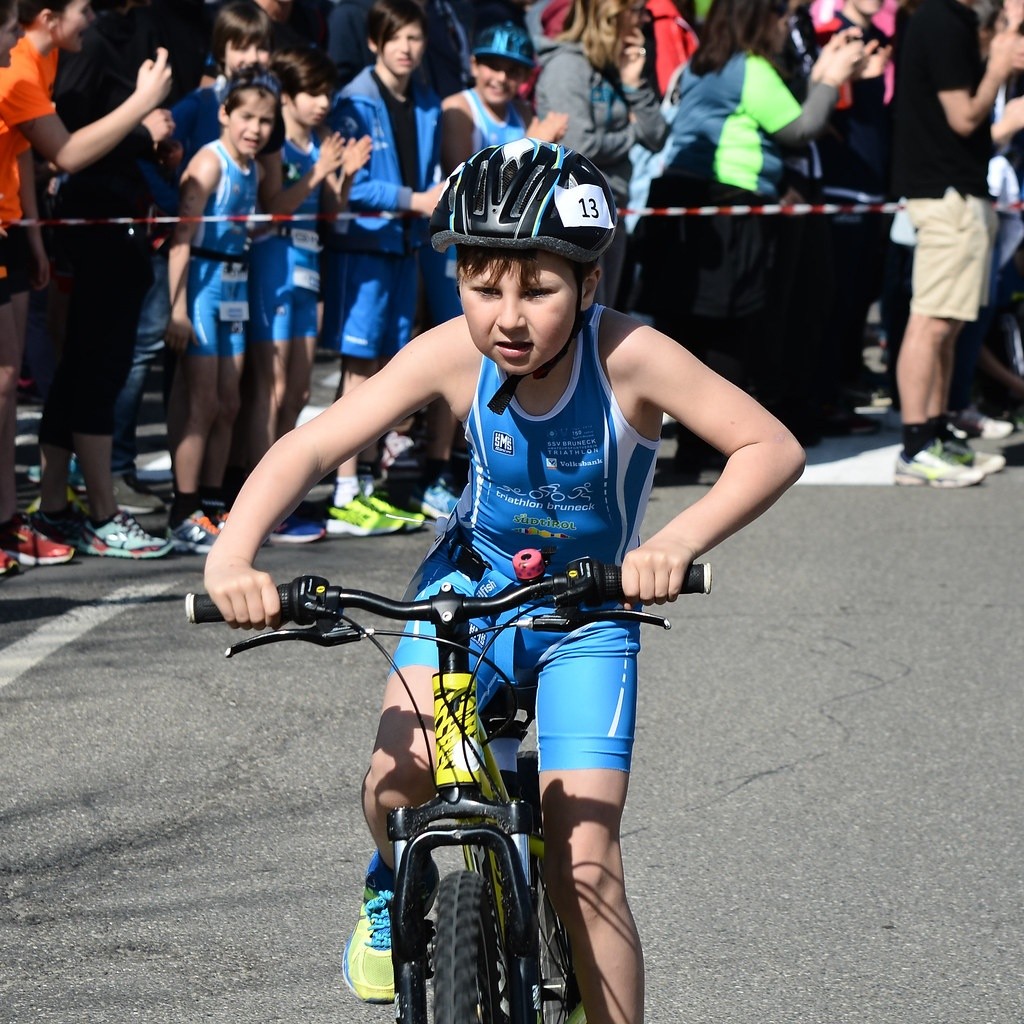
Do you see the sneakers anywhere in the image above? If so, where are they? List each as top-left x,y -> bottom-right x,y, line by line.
935,433 -> 1006,474
203,510 -> 268,545
438,476 -> 462,499
31,511 -> 89,553
325,493 -> 406,537
166,508 -> 221,554
0,522 -> 74,565
361,495 -> 426,530
408,482 -> 462,519
341,850 -> 438,1004
267,514 -> 325,543
0,549 -> 18,575
69,467 -> 163,512
76,512 -> 174,560
894,438 -> 983,489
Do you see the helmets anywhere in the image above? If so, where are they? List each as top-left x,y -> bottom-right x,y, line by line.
429,137 -> 617,263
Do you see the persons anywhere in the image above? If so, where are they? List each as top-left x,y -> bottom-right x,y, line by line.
201,140 -> 808,1024
0,0 -> 1024,577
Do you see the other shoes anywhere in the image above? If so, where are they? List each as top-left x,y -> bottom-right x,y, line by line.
663,443 -> 727,487
948,402 -> 1014,437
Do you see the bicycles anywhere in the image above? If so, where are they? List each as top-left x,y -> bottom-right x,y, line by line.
184,548 -> 711,1024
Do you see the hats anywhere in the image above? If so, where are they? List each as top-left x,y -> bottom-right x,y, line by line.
470,22 -> 537,71
